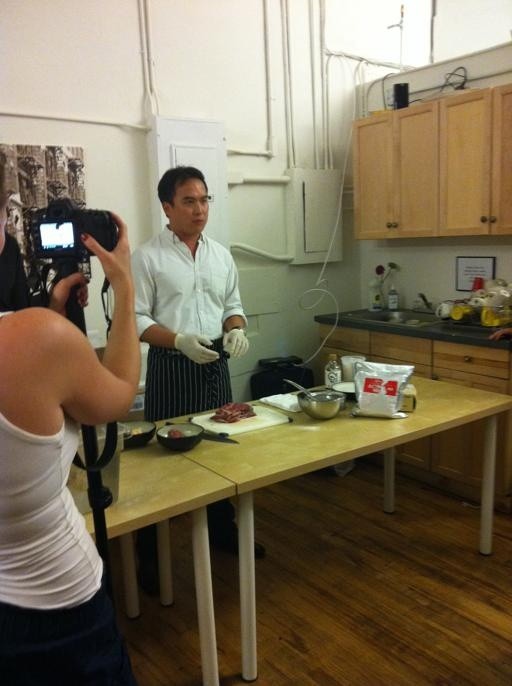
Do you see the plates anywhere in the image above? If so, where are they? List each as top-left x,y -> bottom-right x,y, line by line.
332,383 -> 356,395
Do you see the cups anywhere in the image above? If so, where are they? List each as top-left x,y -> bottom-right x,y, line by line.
341,356 -> 366,382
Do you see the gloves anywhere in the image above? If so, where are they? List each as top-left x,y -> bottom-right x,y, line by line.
222,327 -> 249,362
175,333 -> 219,365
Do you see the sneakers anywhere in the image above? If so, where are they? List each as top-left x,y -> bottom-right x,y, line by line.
212,533 -> 264,558
137,558 -> 161,599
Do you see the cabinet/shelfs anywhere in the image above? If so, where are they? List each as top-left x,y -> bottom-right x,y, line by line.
370,331 -> 431,470
431,332 -> 511,496
318,325 -> 370,387
351,100 -> 439,240
439,83 -> 512,236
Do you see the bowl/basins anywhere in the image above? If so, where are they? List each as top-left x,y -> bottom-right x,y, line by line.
297,391 -> 344,420
156,425 -> 204,451
122,422 -> 155,446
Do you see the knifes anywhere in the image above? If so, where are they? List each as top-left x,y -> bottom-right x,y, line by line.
166,422 -> 239,444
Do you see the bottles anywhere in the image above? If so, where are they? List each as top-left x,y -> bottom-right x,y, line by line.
387,284 -> 400,310
324,354 -> 342,390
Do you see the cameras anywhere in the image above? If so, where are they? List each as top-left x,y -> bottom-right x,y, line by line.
30,200 -> 116,257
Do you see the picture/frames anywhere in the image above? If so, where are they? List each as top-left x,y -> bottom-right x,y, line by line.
455,256 -> 495,291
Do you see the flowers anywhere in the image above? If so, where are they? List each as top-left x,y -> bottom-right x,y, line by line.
376,261 -> 397,282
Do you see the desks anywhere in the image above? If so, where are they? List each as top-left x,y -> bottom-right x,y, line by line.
82,439 -> 236,685
182,385 -> 512,682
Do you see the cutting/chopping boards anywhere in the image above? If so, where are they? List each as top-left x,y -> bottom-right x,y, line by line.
189,406 -> 294,437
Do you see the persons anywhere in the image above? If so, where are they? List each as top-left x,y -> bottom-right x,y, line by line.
106,166 -> 266,583
1,148 -> 141,686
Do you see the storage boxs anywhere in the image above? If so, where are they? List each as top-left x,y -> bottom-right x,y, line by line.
67,421 -> 127,516
